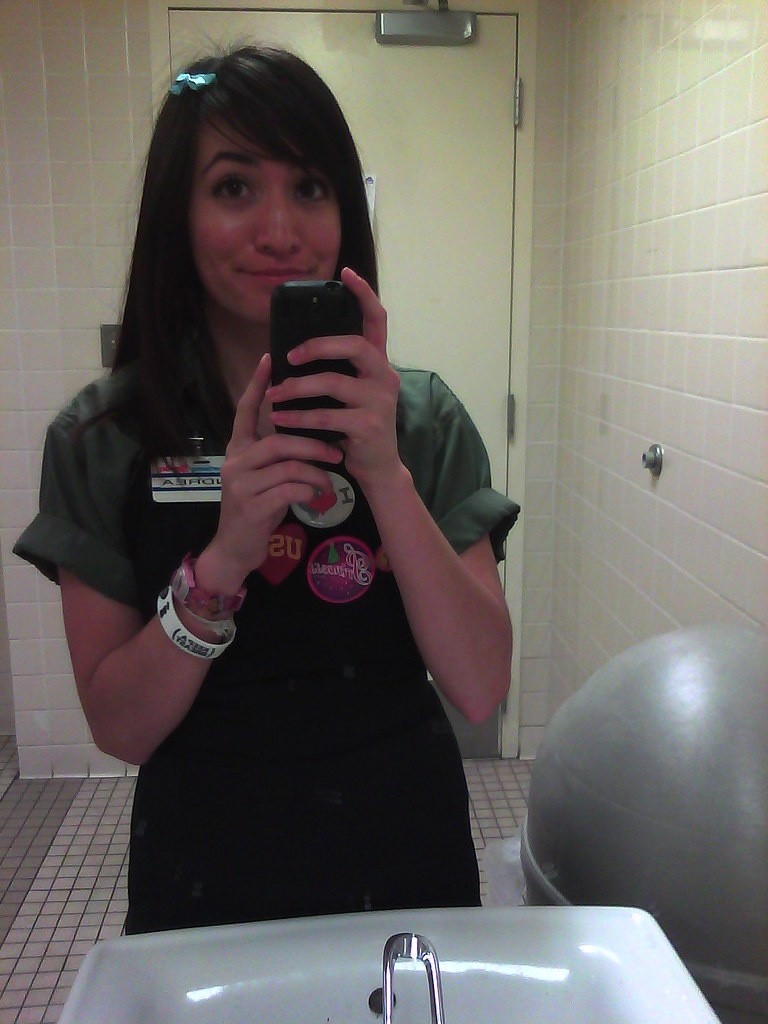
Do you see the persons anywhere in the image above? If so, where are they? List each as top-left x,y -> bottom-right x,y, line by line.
13,34 -> 522,934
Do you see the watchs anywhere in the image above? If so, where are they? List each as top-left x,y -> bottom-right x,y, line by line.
175,553 -> 246,621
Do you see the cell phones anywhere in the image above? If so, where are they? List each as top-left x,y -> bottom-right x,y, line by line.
270,279 -> 358,441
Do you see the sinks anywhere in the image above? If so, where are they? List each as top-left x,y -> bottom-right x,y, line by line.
57,906 -> 720,1024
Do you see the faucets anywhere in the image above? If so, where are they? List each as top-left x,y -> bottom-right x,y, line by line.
384,934 -> 445,1024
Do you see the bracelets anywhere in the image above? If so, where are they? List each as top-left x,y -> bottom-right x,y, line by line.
157,586 -> 237,660
